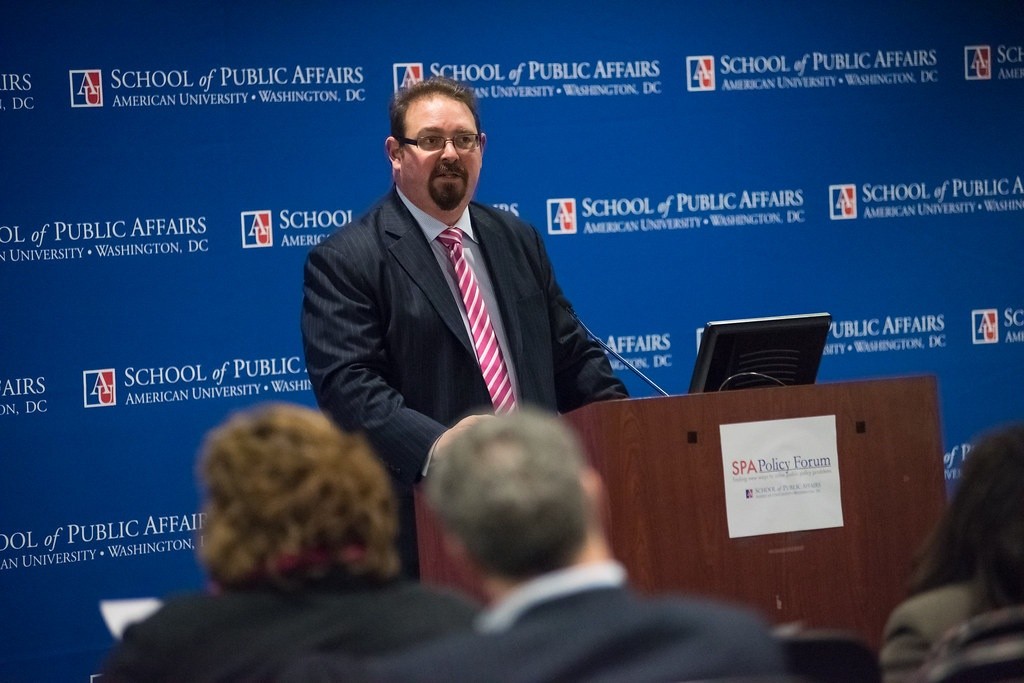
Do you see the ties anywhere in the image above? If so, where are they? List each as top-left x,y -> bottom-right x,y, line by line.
437,227 -> 517,417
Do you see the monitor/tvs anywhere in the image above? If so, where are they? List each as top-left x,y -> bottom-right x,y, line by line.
688,312 -> 832,393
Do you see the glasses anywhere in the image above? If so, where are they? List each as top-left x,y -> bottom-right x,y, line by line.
400,133 -> 482,150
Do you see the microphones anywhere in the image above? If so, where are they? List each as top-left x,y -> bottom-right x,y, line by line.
556,295 -> 671,397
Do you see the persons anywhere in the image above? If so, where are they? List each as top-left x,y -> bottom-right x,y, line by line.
99,407 -> 474,683
300,80 -> 630,593
880,428 -> 1024,683
278,399 -> 790,683
